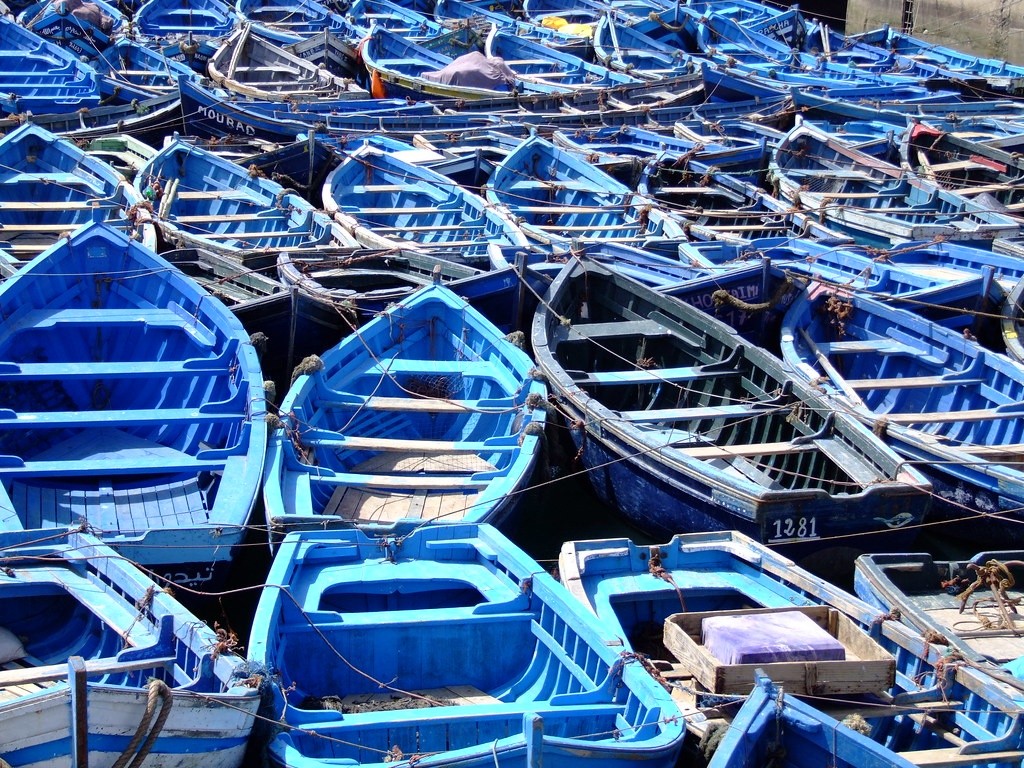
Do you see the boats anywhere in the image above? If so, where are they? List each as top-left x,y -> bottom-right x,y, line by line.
0,201 -> 270,566
853,548 -> 1024,693
261,280 -> 549,566
245,520 -> 687,767
766,283 -> 1024,525
530,249 -> 934,562
706,664 -> 919,768
557,529 -> 1024,768
0,521 -> 262,766
0,0 -> 1024,339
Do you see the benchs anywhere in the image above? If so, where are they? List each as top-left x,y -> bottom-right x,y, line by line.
788,170 -> 965,225
1,310 -> 253,482
0,0 -> 1024,214
503,179 -> 665,242
340,180 -> 503,248
558,310 -> 835,459
809,326 -> 1024,458
282,356 -> 534,490
0,552 -> 1024,768
0,166 -> 135,231
172,182 -> 333,254
656,185 -> 791,232
